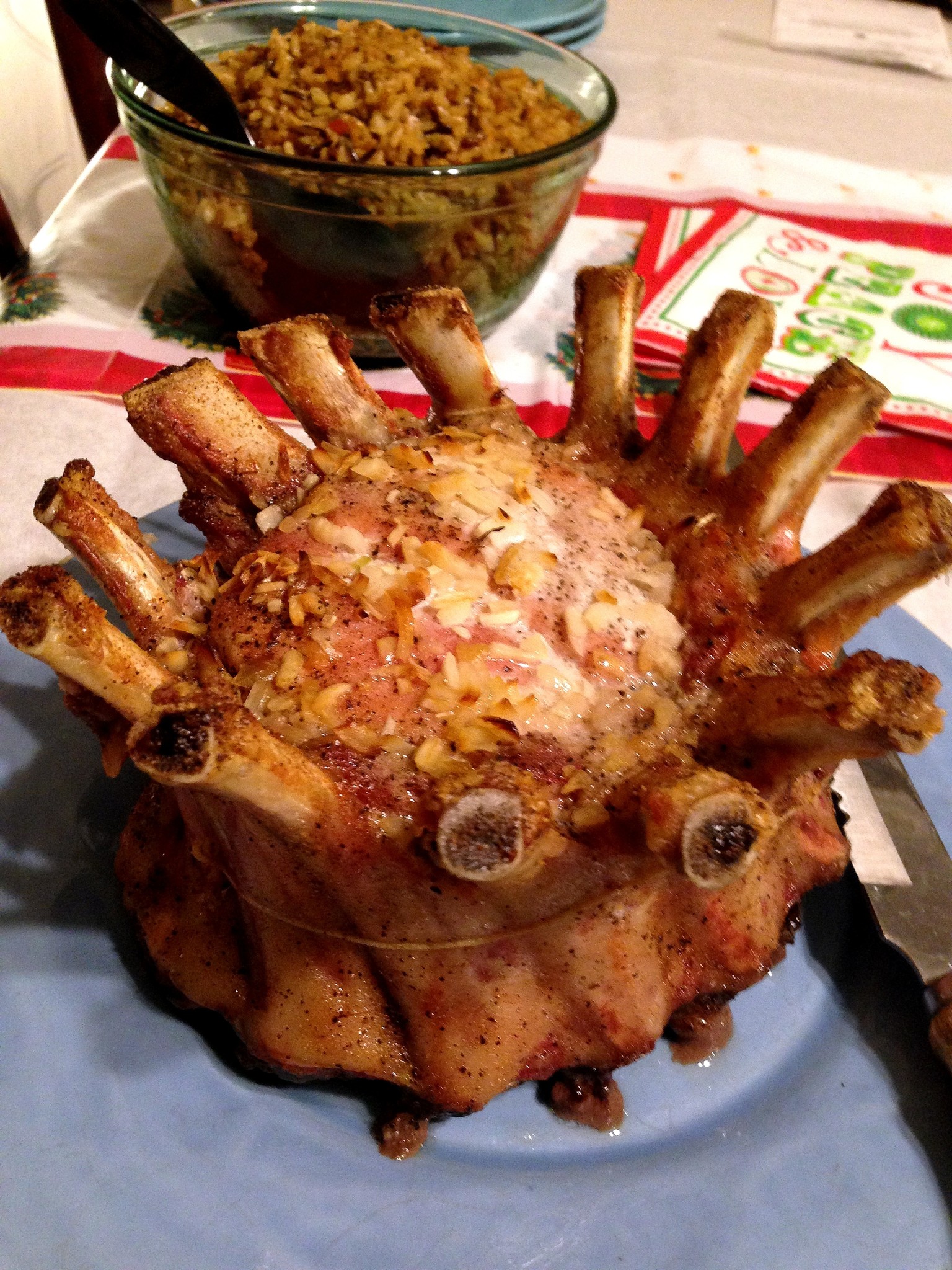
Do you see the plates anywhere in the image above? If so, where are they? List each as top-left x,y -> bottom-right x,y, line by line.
2,472 -> 952,1269
237,1 -> 608,68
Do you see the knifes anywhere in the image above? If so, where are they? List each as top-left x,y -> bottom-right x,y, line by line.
816,636 -> 952,1064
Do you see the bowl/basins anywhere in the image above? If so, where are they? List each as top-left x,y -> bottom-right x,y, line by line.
106,2 -> 624,371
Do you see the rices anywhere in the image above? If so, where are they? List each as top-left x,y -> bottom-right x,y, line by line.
129,10 -> 599,340
